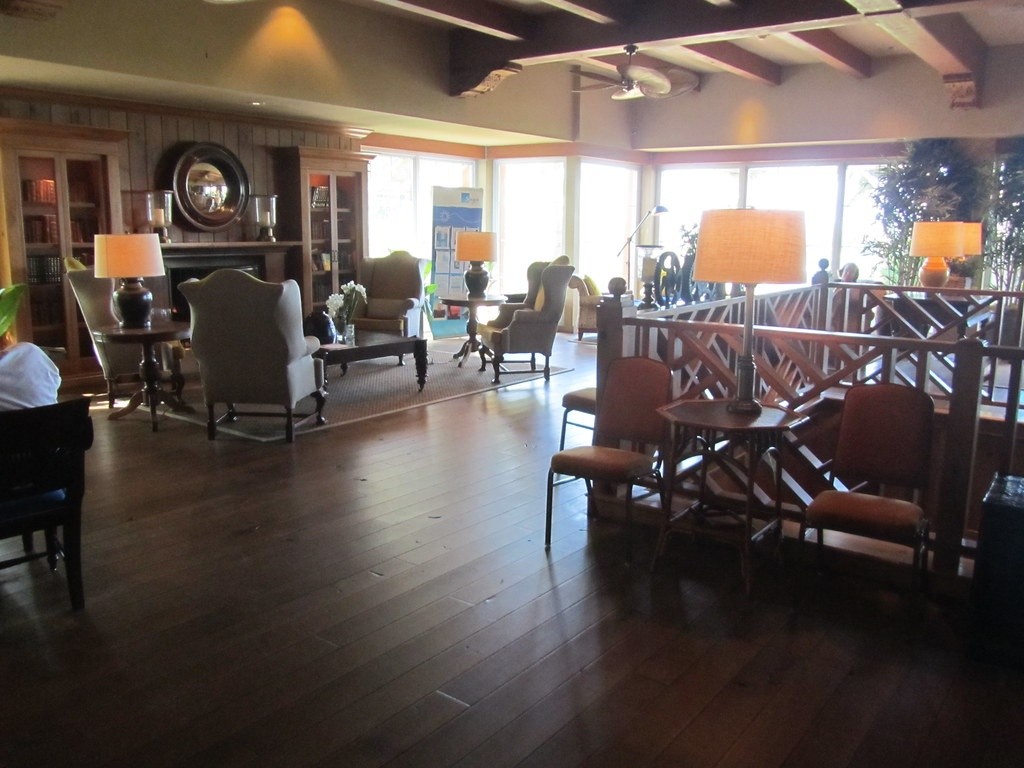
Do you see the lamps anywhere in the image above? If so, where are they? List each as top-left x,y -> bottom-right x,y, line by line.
146,189 -> 173,242
910,220 -> 983,302
457,232 -> 496,298
249,193 -> 279,243
692,208 -> 808,417
92,233 -> 165,331
619,205 -> 670,290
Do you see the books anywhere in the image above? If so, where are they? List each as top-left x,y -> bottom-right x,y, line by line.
311,185 -> 348,240
312,247 -> 354,302
20,179 -> 99,284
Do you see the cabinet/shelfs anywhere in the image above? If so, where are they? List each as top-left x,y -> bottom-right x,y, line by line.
0,118 -> 129,397
296,144 -> 376,320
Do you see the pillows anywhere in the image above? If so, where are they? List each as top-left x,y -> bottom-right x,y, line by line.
583,273 -> 600,295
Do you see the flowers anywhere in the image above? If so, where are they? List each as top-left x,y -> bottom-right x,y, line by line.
327,282 -> 366,316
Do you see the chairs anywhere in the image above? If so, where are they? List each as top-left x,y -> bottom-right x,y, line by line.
0,398 -> 93,613
791,379 -> 942,636
178,269 -> 326,443
544,358 -> 674,557
353,249 -> 433,365
476,254 -> 576,385
568,274 -> 637,342
61,255 -> 186,407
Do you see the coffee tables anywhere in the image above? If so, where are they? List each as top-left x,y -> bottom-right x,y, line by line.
316,330 -> 429,393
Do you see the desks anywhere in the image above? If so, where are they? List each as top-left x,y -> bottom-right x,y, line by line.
657,398 -> 811,608
92,322 -> 194,432
441,298 -> 495,369
884,293 -> 999,403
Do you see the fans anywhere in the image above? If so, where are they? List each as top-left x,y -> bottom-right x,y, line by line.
566,47 -> 701,100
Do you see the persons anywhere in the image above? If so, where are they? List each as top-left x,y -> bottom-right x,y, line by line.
0,328 -> 66,508
832,261 -> 859,304
